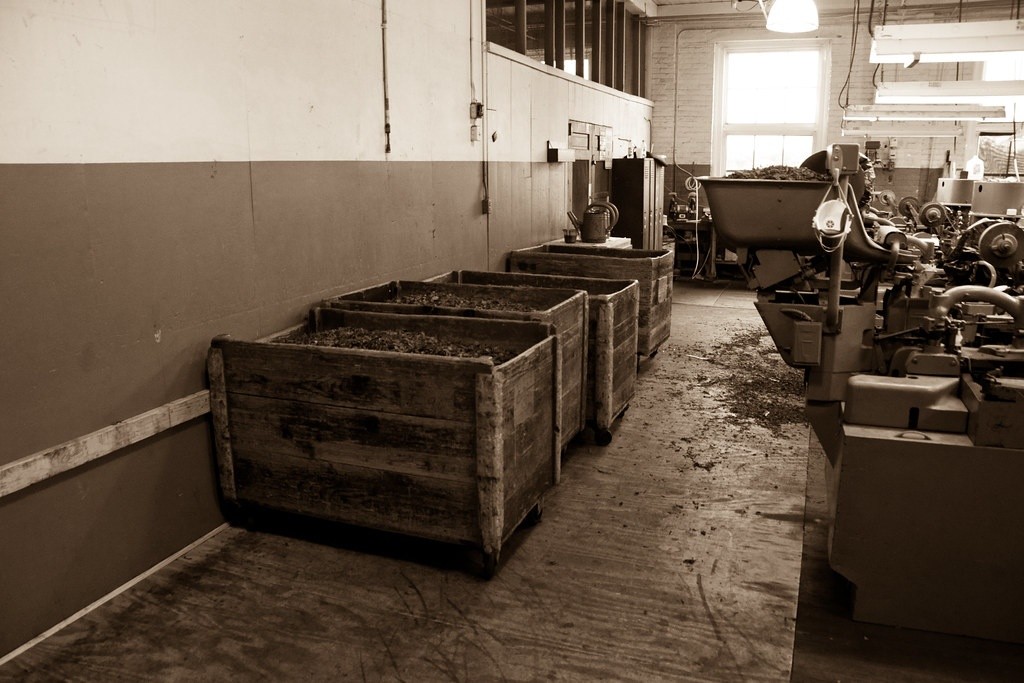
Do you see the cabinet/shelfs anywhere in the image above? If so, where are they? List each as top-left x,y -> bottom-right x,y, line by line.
613,158 -> 665,251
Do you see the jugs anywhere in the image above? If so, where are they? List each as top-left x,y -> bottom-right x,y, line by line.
567,202 -> 619,243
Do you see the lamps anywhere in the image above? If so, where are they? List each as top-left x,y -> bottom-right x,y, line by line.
875,80 -> 1024,108
869,20 -> 1024,63
767,0 -> 819,33
843,105 -> 1005,120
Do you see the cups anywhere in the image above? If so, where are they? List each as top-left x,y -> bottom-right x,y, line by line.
563,229 -> 578,244
